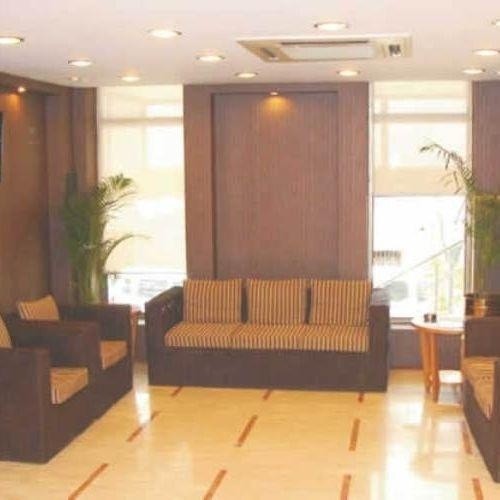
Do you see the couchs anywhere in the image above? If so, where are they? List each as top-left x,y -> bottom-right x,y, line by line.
462,316 -> 500,483
144,278 -> 390,391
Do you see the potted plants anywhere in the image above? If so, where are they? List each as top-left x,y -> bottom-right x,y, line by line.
418,141 -> 500,317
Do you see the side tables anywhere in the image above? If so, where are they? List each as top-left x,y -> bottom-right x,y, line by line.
409,314 -> 465,403
129,308 -> 142,365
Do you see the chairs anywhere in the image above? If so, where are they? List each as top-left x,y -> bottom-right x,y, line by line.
0,293 -> 134,464
